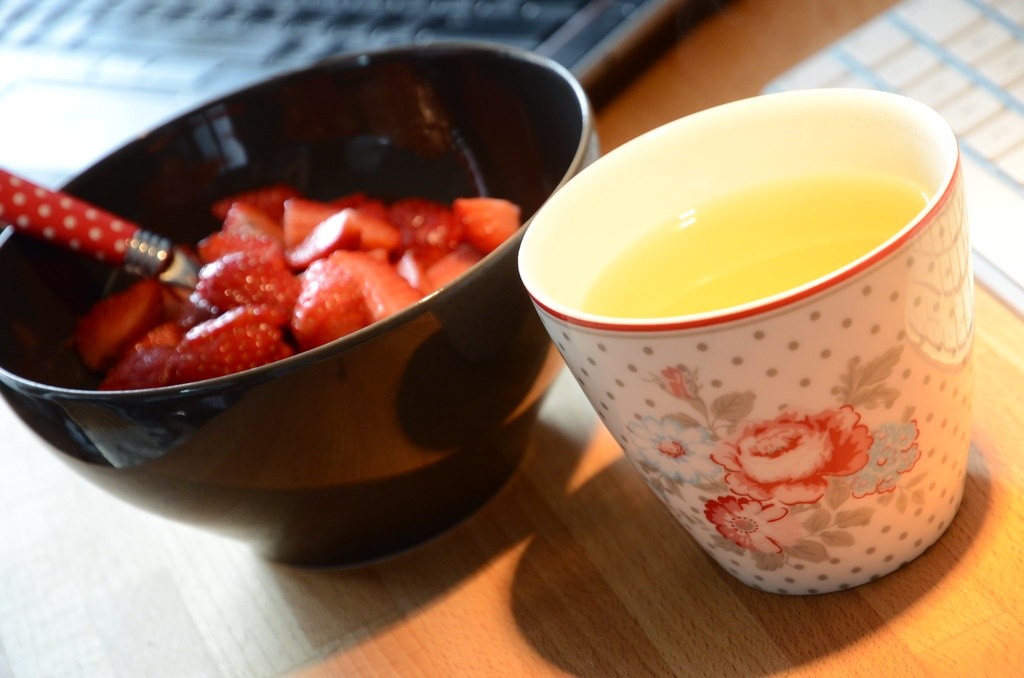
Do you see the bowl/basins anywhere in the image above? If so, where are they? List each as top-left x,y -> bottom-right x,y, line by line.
0,43 -> 600,570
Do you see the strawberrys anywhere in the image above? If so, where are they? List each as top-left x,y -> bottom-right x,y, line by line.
71,182 -> 520,388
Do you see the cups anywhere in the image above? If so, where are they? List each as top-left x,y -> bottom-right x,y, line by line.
518,82 -> 970,593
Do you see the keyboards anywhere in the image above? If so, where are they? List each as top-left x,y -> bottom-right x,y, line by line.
0,0 -> 734,198
